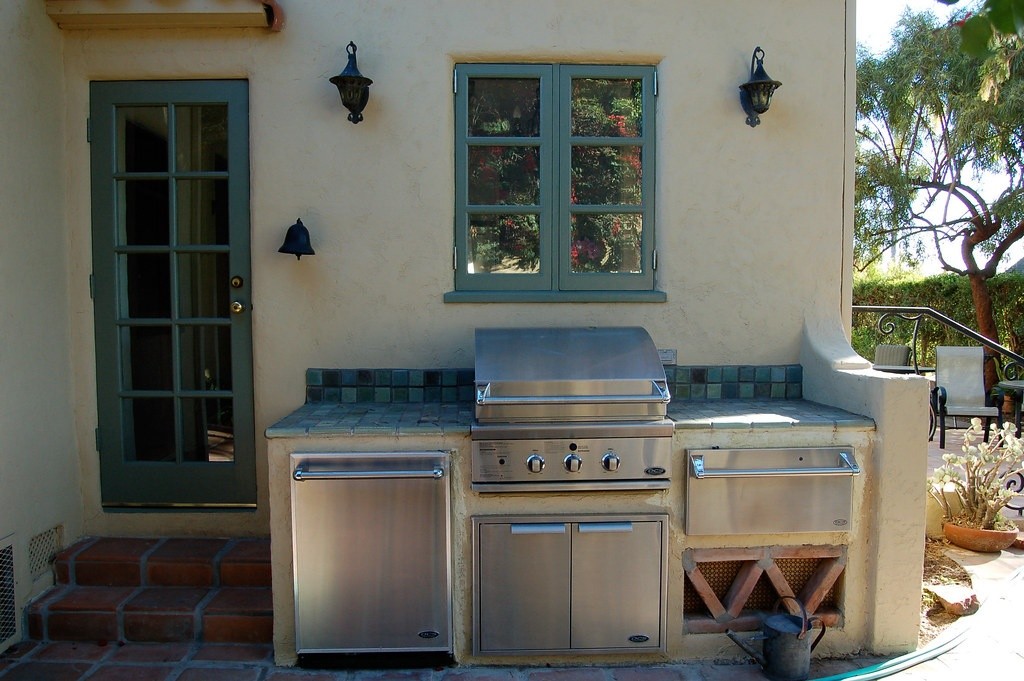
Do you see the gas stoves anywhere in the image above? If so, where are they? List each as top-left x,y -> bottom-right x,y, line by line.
471,328 -> 672,491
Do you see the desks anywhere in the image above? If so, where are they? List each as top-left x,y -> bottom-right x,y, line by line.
998,380 -> 1024,439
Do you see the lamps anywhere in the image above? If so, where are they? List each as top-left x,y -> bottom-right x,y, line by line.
277,218 -> 316,261
328,41 -> 373,124
738,47 -> 782,128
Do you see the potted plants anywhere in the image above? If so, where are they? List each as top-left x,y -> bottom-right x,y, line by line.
991,358 -> 1015,415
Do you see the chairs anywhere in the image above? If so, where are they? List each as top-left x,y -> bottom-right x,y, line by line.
875,344 -> 912,366
931,346 -> 1005,449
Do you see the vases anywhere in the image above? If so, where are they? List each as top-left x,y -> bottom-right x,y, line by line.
927,478 -> 1019,553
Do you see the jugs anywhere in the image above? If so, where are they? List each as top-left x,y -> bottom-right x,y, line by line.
726,596 -> 826,680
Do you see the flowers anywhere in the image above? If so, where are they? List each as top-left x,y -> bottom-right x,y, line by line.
926,418 -> 1024,529
